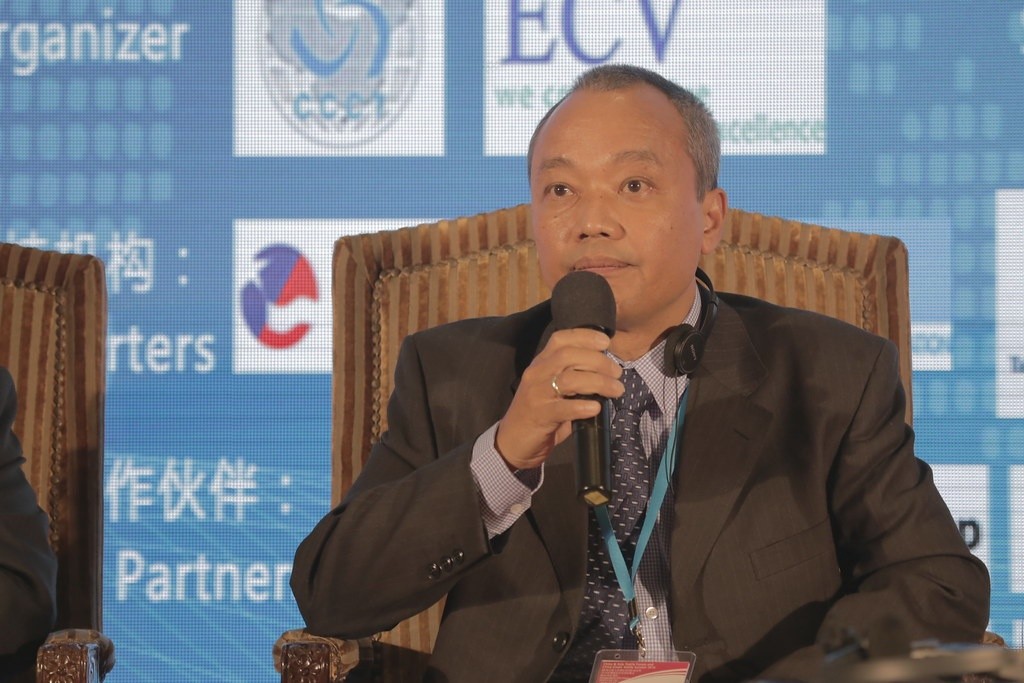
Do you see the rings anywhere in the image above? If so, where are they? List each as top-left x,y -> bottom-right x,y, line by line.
552,371 -> 563,396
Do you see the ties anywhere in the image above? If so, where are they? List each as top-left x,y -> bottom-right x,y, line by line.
561,366 -> 655,671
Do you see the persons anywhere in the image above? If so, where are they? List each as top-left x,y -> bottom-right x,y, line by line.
0,363 -> 56,683
293,65 -> 990,683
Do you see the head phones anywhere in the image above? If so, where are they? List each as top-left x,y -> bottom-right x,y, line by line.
665,267 -> 719,377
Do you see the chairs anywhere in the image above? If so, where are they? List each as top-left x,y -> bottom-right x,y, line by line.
0,243 -> 116,683
274,205 -> 1007,683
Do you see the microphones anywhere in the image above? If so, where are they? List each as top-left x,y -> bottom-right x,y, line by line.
551,270 -> 616,506
